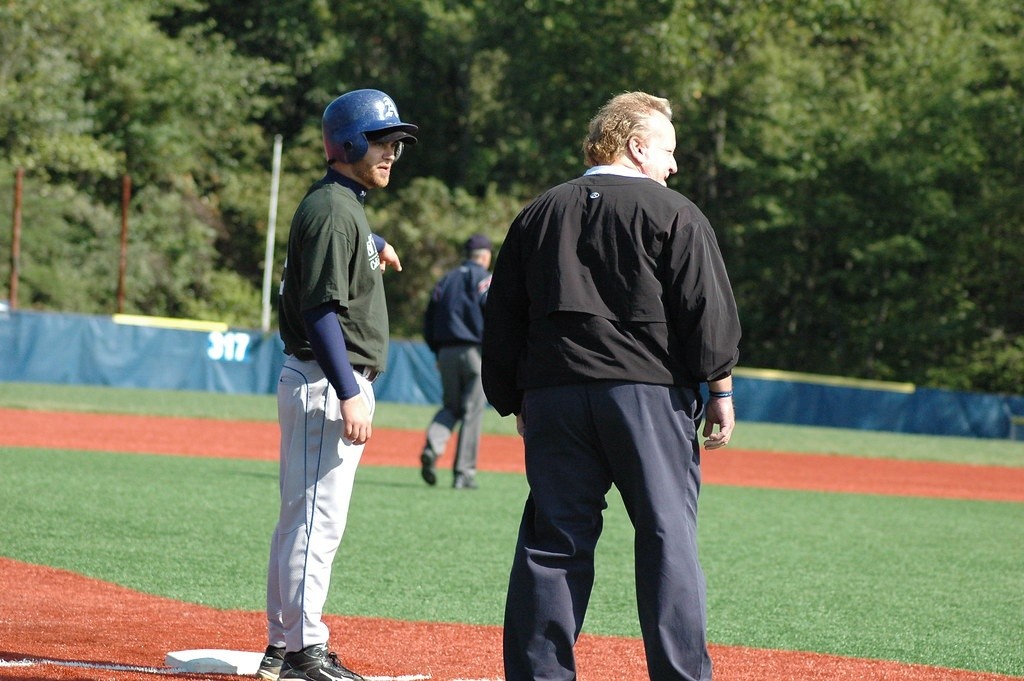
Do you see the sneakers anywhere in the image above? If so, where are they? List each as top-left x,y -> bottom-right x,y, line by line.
278,642 -> 366,681
256,645 -> 288,681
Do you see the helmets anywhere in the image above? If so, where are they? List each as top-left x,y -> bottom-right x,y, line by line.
322,90 -> 419,164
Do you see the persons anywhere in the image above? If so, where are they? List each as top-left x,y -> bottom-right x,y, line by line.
257,89 -> 417,680
482,92 -> 742,680
420,235 -> 493,490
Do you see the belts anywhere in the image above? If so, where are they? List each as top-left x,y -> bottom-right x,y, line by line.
286,339 -> 377,381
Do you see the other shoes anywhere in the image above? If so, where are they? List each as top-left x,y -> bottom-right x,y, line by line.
453,471 -> 479,487
420,448 -> 437,484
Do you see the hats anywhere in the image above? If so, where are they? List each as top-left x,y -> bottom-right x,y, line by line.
368,128 -> 418,146
464,233 -> 491,250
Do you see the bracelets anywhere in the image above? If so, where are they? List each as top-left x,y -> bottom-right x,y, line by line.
710,391 -> 734,397
